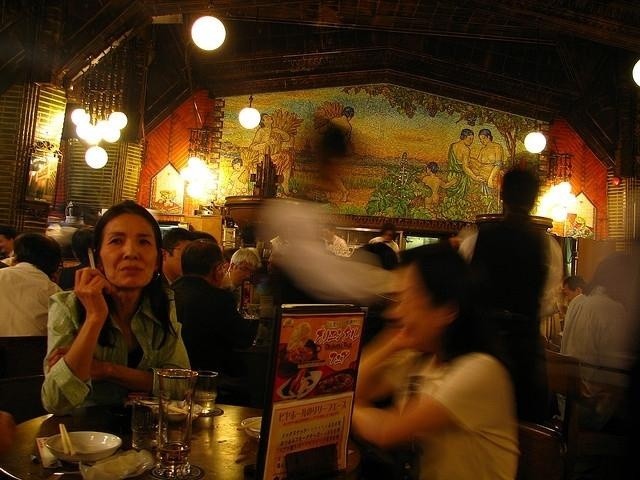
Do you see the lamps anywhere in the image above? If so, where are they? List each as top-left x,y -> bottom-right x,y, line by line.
190,0 -> 227,52
632,59 -> 640,87
69,34 -> 129,170
524,120 -> 547,154
238,63 -> 261,129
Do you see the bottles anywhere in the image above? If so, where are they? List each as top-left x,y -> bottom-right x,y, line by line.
221,206 -> 237,251
96,208 -> 102,224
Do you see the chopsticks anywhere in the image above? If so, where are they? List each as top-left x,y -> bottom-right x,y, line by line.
237,419 -> 261,431
165,404 -> 198,419
58,423 -> 76,456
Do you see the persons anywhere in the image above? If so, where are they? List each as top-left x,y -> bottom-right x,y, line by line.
0,200 -> 638,479
228,105 -> 504,219
244,117 -> 399,408
457,167 -> 563,425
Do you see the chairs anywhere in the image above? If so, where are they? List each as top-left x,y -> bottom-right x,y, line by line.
516,408 -> 570,479
0,335 -> 47,426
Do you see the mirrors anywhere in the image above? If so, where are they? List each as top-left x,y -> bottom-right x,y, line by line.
22,139 -> 64,216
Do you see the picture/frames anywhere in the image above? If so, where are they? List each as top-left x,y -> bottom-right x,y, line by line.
563,190 -> 597,241
149,161 -> 185,216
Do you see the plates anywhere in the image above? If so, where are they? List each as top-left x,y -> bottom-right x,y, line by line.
276,339 -> 357,400
156,400 -> 205,422
240,416 -> 263,438
44,432 -> 124,463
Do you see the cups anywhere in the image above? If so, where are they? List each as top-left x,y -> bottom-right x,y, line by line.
155,368 -> 198,477
131,395 -> 168,433
193,370 -> 219,415
